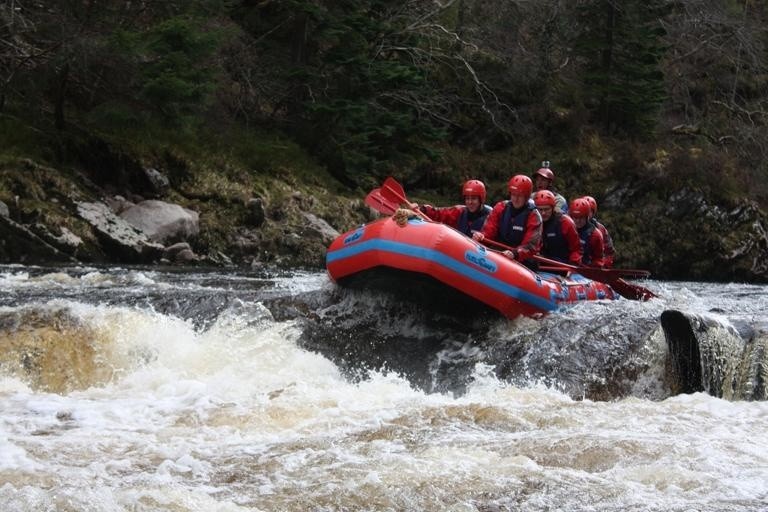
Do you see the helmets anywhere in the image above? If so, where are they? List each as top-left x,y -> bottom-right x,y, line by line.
461,179 -> 487,204
568,195 -> 597,218
534,189 -> 557,210
507,174 -> 533,200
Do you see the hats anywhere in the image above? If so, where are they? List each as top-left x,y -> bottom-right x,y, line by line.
532,168 -> 554,181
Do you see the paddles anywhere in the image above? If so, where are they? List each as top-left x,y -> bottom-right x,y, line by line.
485,238 -> 658,301
364,188 -> 400,216
380,177 -> 431,221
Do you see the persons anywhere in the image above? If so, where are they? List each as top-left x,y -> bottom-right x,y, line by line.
527,167 -> 570,216
535,188 -> 585,276
566,197 -> 605,269
578,195 -> 617,269
471,174 -> 544,273
408,178 -> 494,238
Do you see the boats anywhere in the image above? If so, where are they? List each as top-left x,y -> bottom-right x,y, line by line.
326,214 -> 617,320
